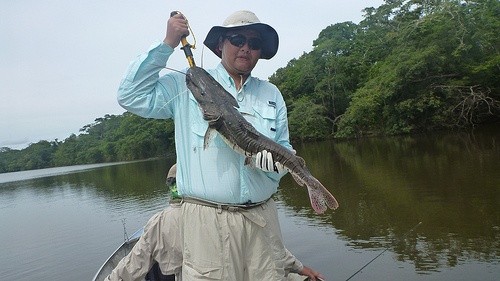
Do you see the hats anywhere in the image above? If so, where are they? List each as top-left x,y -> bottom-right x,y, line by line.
166,163 -> 177,178
203,7 -> 280,60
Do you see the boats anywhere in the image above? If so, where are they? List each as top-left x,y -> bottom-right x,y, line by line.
91,223 -> 327,281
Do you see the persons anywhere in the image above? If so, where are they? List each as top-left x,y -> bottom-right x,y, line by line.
117,11 -> 297,281
104,162 -> 326,281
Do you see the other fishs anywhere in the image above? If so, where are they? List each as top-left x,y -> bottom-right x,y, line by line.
184,65 -> 339,214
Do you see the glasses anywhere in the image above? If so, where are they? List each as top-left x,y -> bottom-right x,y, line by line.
222,32 -> 264,53
